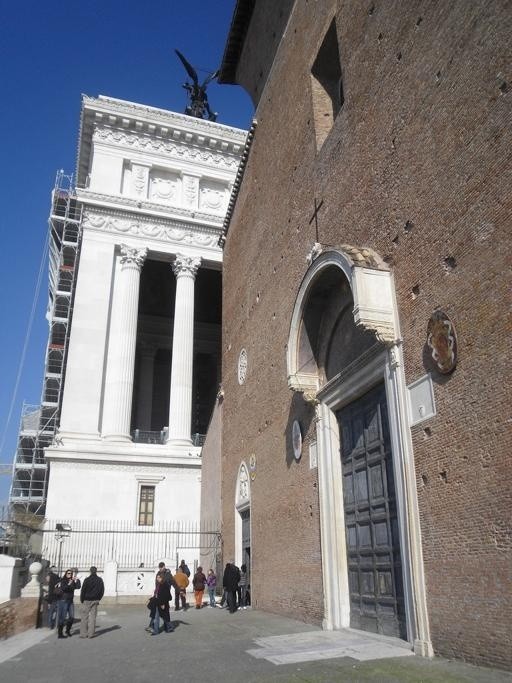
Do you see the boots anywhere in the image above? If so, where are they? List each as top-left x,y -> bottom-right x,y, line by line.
58,625 -> 67,638
65,622 -> 72,636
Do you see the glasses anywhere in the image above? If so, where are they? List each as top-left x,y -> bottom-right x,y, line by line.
68,572 -> 73,575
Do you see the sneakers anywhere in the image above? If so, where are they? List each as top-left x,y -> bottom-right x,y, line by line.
215,604 -> 222,609
237,606 -> 241,610
243,606 -> 247,610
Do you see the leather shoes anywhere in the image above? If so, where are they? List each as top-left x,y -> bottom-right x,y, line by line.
145,627 -> 173,635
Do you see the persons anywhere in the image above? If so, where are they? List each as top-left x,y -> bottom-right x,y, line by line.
79,566 -> 103,638
48,566 -> 62,629
138,563 -> 144,589
145,559 -> 249,635
55,570 -> 80,638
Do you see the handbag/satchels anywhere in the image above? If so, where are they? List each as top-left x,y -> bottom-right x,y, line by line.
52,586 -> 64,599
